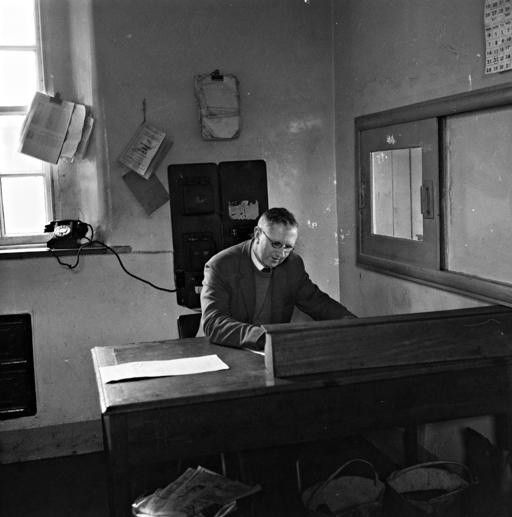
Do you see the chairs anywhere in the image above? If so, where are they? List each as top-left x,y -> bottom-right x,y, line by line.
176,310 -> 208,337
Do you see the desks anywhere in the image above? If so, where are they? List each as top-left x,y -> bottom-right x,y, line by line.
90,307 -> 510,517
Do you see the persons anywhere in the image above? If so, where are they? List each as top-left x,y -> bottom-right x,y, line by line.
193,204 -> 359,350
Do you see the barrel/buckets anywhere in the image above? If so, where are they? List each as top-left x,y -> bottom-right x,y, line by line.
301,458 -> 386,516
385,460 -> 479,516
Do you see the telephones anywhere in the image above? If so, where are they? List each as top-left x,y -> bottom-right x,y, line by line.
44,220 -> 88,249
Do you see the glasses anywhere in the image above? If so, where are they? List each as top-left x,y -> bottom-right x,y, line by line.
260,228 -> 295,254
